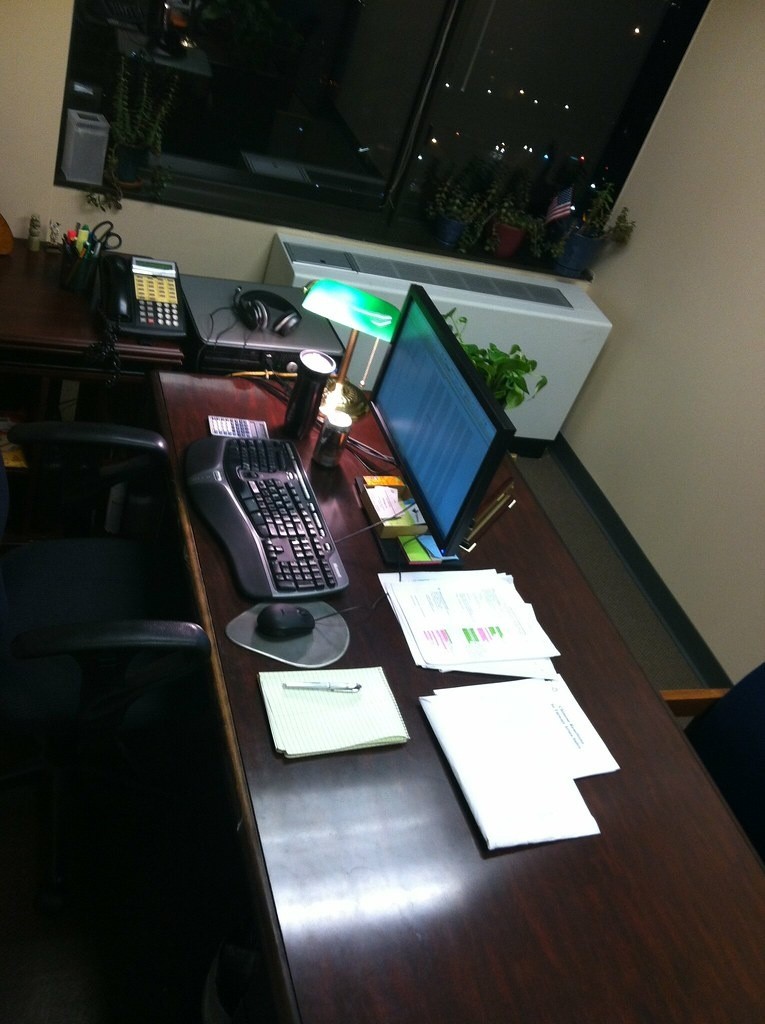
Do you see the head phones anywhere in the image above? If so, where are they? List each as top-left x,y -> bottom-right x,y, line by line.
239,290 -> 303,337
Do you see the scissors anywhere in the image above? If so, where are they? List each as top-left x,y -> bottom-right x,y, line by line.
91,221 -> 122,249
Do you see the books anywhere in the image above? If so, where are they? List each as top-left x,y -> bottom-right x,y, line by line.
258,666 -> 412,758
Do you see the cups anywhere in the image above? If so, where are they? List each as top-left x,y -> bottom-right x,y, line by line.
281,348 -> 338,441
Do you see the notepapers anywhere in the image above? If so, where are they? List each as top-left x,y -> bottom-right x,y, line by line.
397,535 -> 442,564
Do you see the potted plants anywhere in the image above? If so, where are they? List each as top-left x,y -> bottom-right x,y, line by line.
426,157 -> 497,248
485,165 -> 544,262
85,56 -> 181,214
548,181 -> 635,278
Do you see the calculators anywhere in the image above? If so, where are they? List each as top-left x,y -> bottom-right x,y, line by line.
208,415 -> 269,439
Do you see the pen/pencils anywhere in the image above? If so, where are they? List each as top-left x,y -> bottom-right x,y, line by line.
285,683 -> 361,693
62,222 -> 101,257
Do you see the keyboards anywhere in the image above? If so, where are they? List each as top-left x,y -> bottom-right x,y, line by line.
183,437 -> 350,603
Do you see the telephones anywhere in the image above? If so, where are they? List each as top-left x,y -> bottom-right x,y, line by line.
100,254 -> 188,339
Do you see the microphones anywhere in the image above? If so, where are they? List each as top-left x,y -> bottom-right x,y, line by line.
233,287 -> 251,321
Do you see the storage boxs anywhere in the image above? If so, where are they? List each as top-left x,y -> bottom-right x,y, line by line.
262,232 -> 614,462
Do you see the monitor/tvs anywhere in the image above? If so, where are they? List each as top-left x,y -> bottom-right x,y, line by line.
367,284 -> 516,556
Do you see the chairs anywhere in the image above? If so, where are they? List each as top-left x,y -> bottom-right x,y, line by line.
659,663 -> 764,869
0,417 -> 210,782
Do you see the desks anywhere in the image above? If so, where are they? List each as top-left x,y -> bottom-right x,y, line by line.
1,235 -> 289,446
158,357 -> 764,1024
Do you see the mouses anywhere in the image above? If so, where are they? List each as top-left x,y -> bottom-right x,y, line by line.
257,604 -> 315,640
